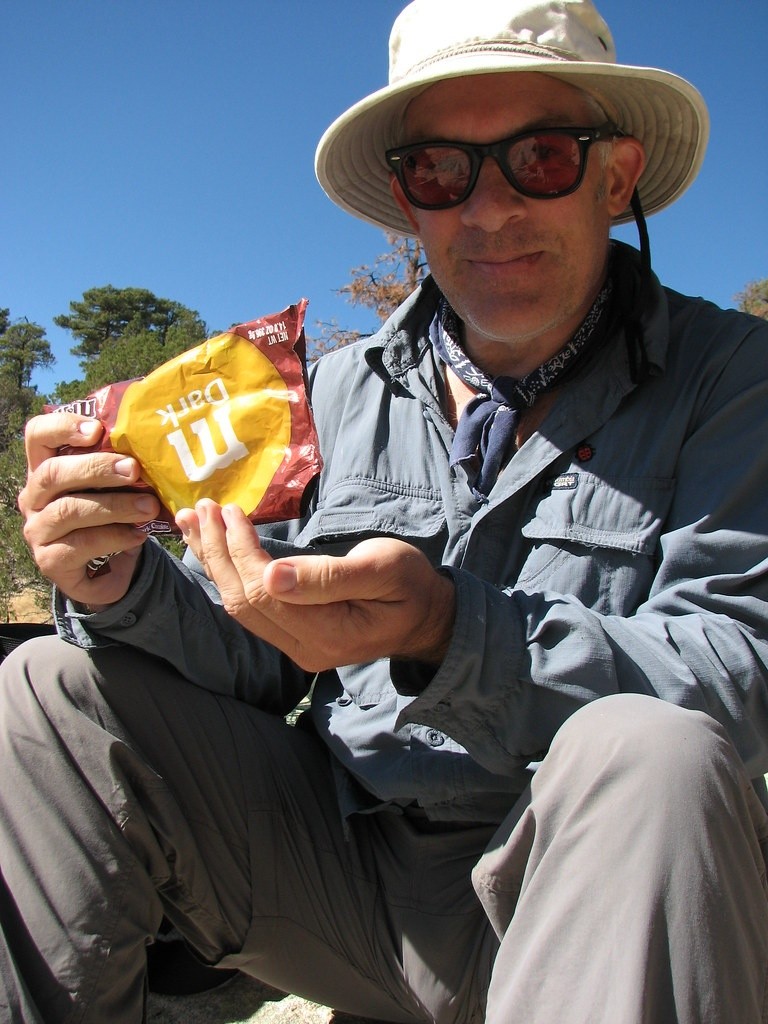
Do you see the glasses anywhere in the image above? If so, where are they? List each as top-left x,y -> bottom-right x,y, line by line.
384,127 -> 617,210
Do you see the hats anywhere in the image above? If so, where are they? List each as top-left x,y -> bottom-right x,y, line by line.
313,0 -> 708,225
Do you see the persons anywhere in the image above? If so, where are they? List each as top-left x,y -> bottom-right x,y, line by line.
0,0 -> 768,1024
